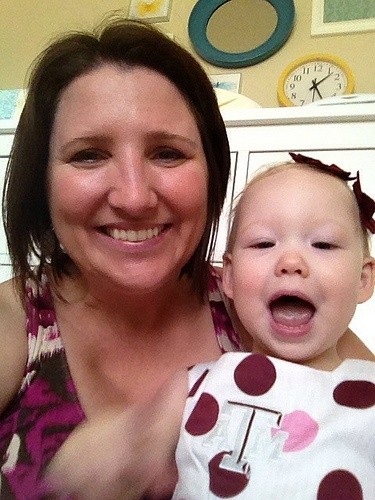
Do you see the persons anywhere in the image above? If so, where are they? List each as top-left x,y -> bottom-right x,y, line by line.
0,18 -> 375,500
44,161 -> 375,500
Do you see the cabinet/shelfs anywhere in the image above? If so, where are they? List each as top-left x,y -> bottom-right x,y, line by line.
1,109 -> 375,287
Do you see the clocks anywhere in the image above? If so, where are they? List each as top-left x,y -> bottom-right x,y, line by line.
276,52 -> 356,107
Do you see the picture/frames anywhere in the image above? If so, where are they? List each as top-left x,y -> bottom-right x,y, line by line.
207,72 -> 241,95
310,0 -> 375,38
126,0 -> 173,23
